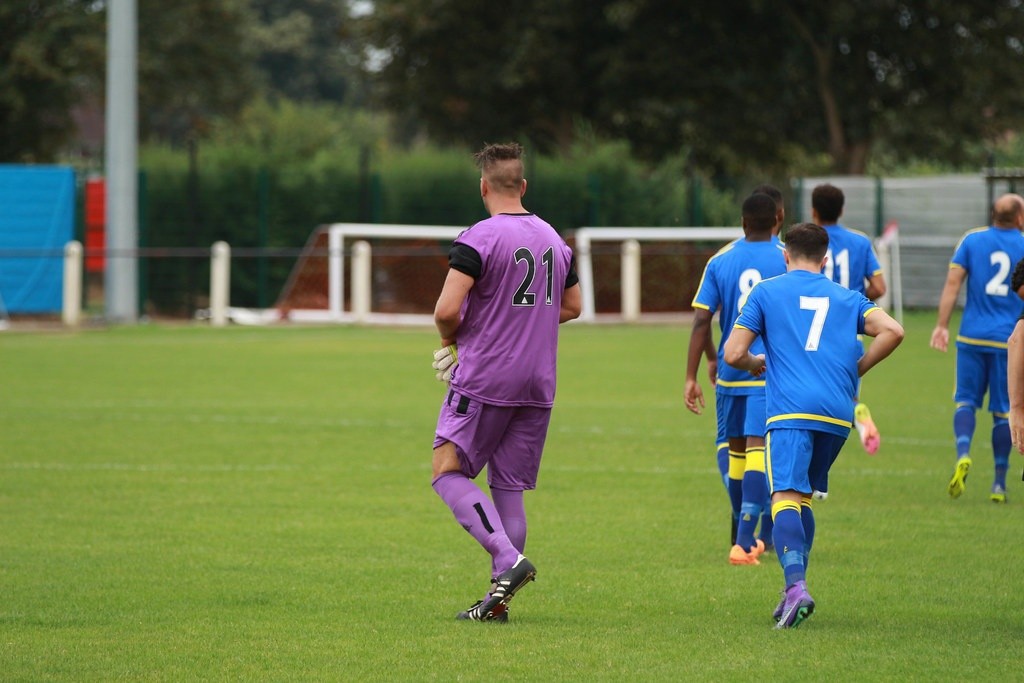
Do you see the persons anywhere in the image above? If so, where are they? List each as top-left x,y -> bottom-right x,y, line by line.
808,182 -> 890,456
722,222 -> 905,630
681,185 -> 787,568
1007,256 -> 1024,301
1006,312 -> 1024,457
425,144 -> 582,624
927,192 -> 1024,503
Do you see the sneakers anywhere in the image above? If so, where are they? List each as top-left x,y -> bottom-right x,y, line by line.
729,544 -> 761,566
772,578 -> 816,628
990,483 -> 1008,503
853,402 -> 881,456
750,538 -> 765,558
479,553 -> 537,620
947,454 -> 973,499
455,600 -> 509,622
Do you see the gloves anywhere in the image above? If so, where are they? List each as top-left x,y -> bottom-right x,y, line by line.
432,342 -> 459,383
